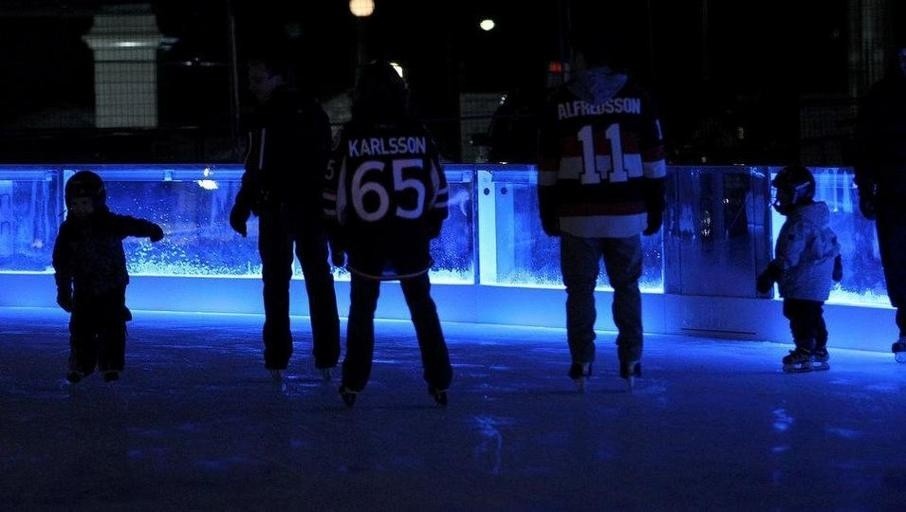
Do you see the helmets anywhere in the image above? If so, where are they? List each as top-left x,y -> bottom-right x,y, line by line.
768,166 -> 815,217
65,170 -> 107,224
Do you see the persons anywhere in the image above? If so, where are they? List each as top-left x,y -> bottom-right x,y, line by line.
52,171 -> 164,382
228,56 -> 341,368
324,60 -> 454,392
758,166 -> 844,361
853,33 -> 905,350
540,35 -> 668,378
484,75 -> 549,162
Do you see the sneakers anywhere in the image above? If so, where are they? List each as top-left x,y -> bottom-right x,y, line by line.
891,335 -> 906,353
264,355 -> 289,369
783,349 -> 813,363
315,354 -> 337,368
622,361 -> 641,379
65,369 -> 84,385
814,347 -> 828,362
103,371 -> 120,382
424,366 -> 453,394
573,361 -> 592,377
343,369 -> 367,394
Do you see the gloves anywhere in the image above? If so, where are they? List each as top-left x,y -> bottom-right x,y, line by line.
832,256 -> 843,282
642,212 -> 664,235
140,220 -> 164,242
756,266 -> 777,295
426,212 -> 444,239
329,242 -> 345,267
230,203 -> 249,237
858,191 -> 873,216
540,206 -> 560,239
57,290 -> 73,312
